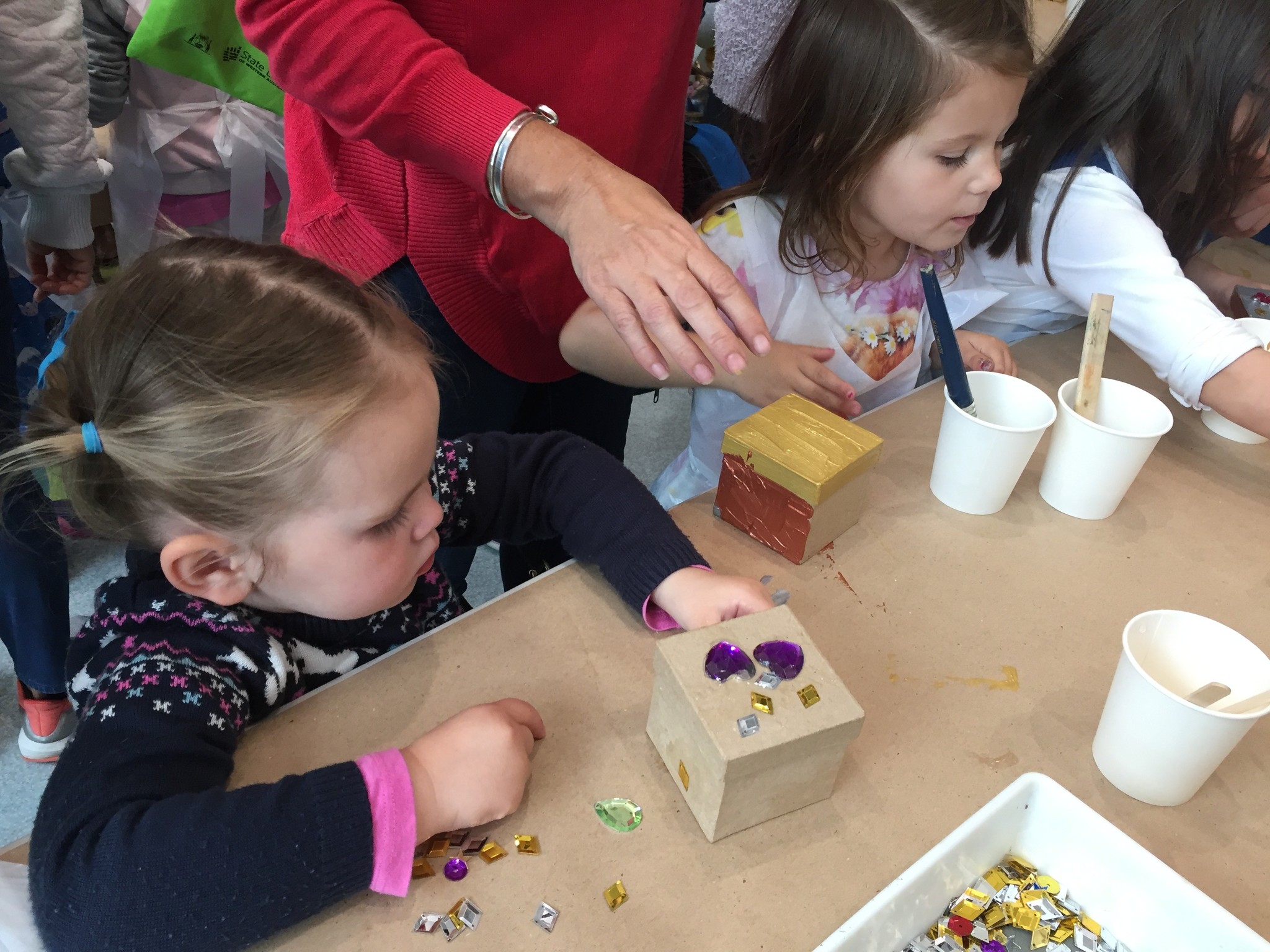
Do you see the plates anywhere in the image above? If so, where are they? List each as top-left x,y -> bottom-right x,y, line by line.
811,770 -> 1270,952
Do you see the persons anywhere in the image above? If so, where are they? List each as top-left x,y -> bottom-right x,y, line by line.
0,238 -> 782,950
558,0 -> 1270,512
233,0 -> 706,598
79,0 -> 291,270
0,0 -> 105,764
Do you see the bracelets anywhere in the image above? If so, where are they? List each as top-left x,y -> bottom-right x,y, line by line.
486,102 -> 560,222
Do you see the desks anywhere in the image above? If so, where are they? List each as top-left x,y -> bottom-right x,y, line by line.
1,237 -> 1269,950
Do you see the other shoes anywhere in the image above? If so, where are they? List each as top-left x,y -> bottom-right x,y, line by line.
17,680 -> 79,763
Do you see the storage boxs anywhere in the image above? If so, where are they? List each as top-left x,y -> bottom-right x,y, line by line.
809,771 -> 1270,952
645,605 -> 866,843
713,392 -> 885,566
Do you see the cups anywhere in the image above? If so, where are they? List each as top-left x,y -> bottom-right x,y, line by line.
1091,610 -> 1270,808
927,371 -> 1057,515
1201,318 -> 1270,446
1037,377 -> 1173,521
